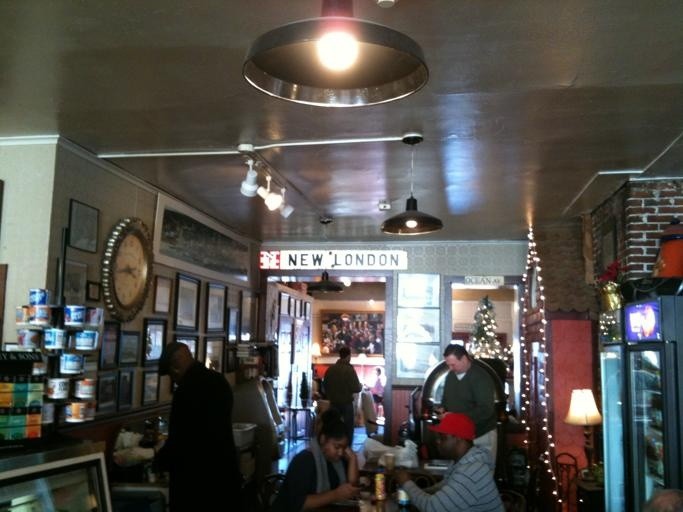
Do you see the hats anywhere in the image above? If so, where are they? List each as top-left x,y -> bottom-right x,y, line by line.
427,414 -> 475,440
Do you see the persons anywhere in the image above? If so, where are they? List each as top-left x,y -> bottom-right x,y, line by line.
324,347 -> 362,447
274,409 -> 359,512
443,344 -> 498,468
114,342 -> 239,511
398,413 -> 506,512
364,368 -> 385,403
322,313 -> 383,354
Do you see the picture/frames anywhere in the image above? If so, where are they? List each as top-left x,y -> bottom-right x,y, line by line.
203,280 -> 228,335
172,271 -> 202,330
86,281 -> 101,303
97,368 -> 117,412
117,329 -> 141,367
151,193 -> 254,290
100,318 -> 120,369
319,309 -> 385,357
276,290 -> 310,324
62,198 -> 100,254
174,334 -> 200,363
238,289 -> 259,342
142,316 -> 168,366
64,258 -> 88,303
141,368 -> 161,407
227,306 -> 239,344
115,368 -> 135,410
202,337 -> 225,373
153,274 -> 174,312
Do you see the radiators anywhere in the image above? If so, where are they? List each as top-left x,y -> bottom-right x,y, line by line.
391,389 -> 421,446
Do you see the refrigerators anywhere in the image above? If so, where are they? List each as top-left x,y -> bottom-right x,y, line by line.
623,295 -> 683,512
599,310 -> 625,512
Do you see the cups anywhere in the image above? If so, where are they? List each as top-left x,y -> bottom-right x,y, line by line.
385,453 -> 395,471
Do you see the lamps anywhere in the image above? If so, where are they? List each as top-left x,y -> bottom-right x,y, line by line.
237,144 -> 295,219
308,217 -> 344,294
380,133 -> 445,236
241,0 -> 430,108
